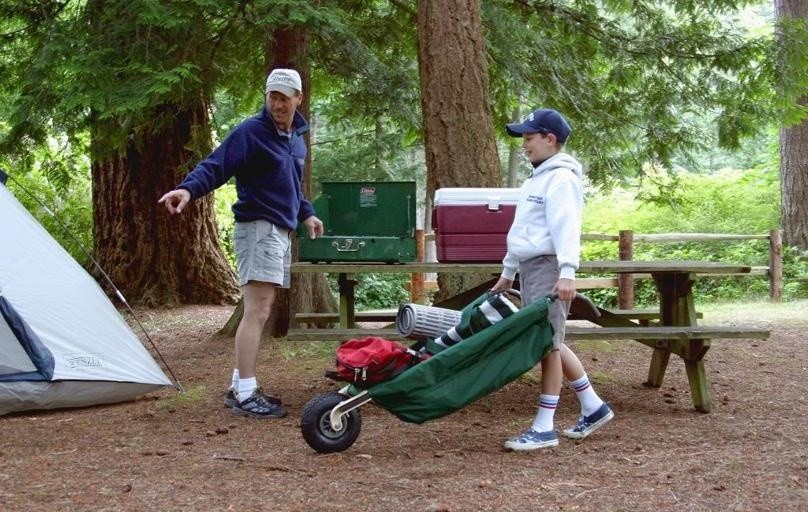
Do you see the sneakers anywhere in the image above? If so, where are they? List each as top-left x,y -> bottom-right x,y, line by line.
502,428 -> 562,451
223,387 -> 282,411
563,401 -> 615,440
232,391 -> 288,420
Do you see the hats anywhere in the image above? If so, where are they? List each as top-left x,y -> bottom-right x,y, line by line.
263,68 -> 305,99
504,107 -> 573,147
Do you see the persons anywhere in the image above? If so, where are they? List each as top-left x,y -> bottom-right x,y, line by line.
490,108 -> 615,452
157,68 -> 324,418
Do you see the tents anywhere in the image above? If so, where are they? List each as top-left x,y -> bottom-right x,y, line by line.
0,171 -> 172,417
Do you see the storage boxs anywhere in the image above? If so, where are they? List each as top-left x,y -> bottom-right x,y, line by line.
429,185 -> 524,260
290,176 -> 421,266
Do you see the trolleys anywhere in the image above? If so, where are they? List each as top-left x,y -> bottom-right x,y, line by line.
299,288 -> 602,454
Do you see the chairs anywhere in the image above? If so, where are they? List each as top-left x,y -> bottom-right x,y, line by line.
285,262 -> 771,414
